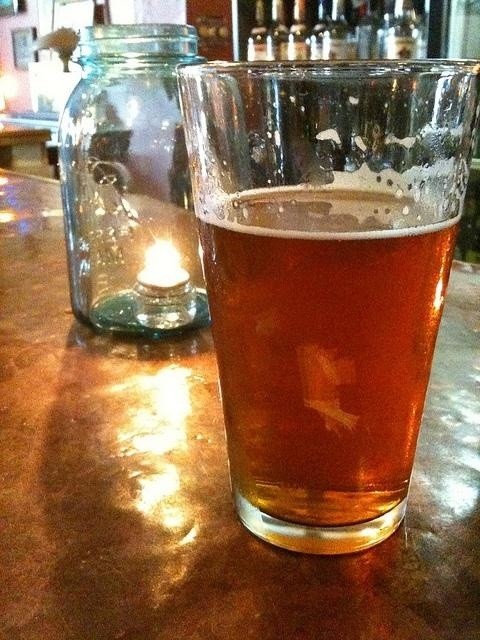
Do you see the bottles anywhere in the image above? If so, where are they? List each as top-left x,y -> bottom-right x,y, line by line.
270,0 -> 288,62
310,1 -> 330,60
246,0 -> 270,60
288,0 -> 310,60
56,21 -> 223,344
330,0 -> 428,61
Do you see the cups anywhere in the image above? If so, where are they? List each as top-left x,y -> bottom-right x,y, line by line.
173,59 -> 479,560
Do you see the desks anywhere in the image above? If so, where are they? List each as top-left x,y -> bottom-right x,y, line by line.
1,168 -> 478,637
1,123 -> 52,179
